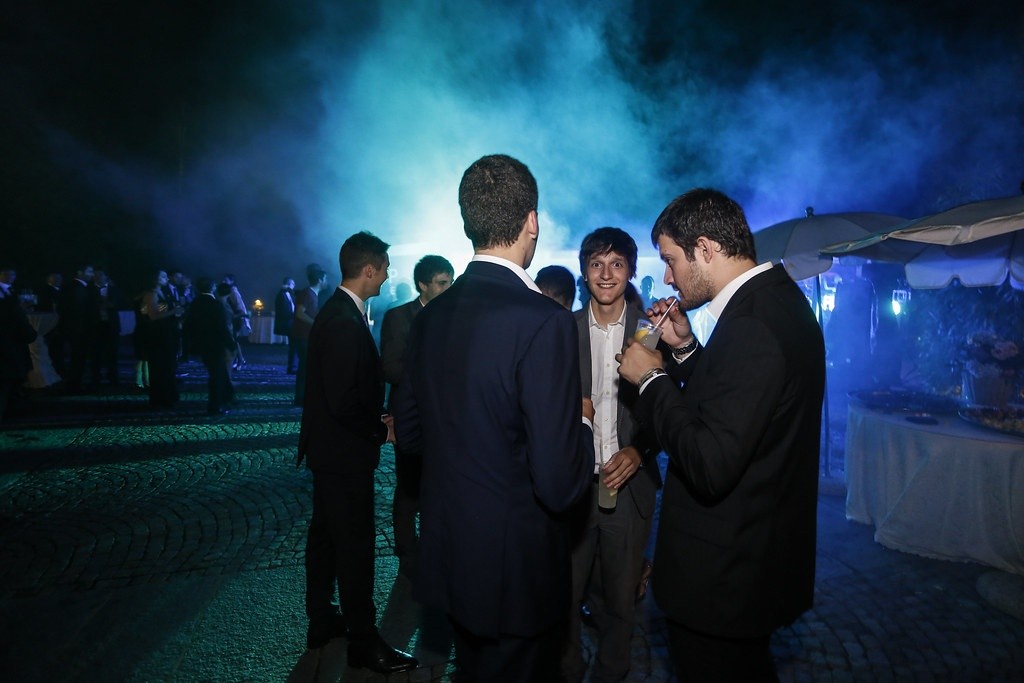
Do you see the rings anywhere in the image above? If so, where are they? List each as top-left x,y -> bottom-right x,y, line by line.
675,305 -> 680,311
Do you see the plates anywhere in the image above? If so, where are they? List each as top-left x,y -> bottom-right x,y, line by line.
957,406 -> 1024,434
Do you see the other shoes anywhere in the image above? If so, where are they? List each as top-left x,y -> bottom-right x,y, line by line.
131,383 -> 151,394
393,541 -> 419,558
208,407 -> 230,414
581,606 -> 598,631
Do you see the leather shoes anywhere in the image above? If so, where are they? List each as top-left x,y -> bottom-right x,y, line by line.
348,638 -> 420,675
306,619 -> 346,651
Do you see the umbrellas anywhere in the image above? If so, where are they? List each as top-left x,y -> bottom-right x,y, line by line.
737,206 -> 921,331
818,193 -> 1024,294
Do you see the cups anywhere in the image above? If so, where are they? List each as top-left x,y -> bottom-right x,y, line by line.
598,463 -> 617,509
634,319 -> 664,352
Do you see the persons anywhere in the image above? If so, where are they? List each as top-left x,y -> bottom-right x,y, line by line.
615,187 -> 828,683
635,276 -> 659,313
391,151 -> 598,682
576,275 -> 592,308
294,231 -> 421,676
1,257 -> 329,419
551,227 -> 683,683
834,264 -> 879,365
529,265 -> 578,313
381,255 -> 457,580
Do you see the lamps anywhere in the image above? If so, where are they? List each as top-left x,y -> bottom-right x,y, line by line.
252,299 -> 264,316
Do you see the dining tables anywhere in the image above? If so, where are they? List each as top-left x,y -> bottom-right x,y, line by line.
249,313 -> 290,345
848,391 -> 1023,578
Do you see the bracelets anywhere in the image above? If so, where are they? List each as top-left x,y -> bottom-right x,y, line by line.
667,336 -> 699,355
637,367 -> 667,390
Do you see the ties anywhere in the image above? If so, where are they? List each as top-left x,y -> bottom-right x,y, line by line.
96,286 -> 106,296
